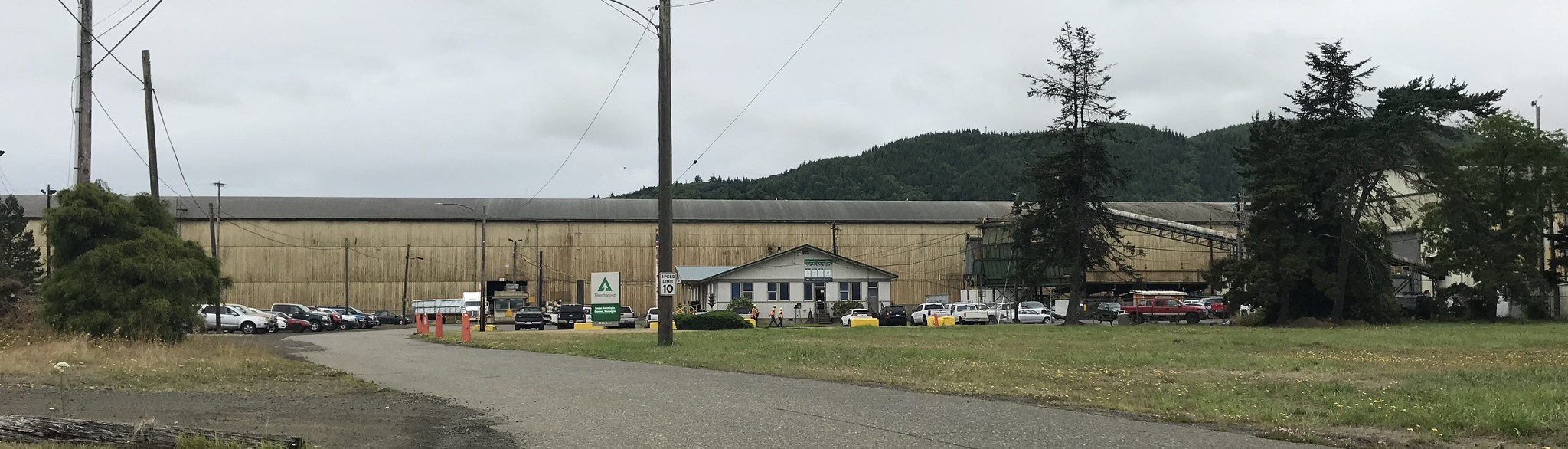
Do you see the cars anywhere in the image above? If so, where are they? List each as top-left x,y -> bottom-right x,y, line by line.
1010,308 -> 1055,324
232,306 -> 312,333
840,308 -> 872,327
374,312 -> 412,325
540,300 -> 592,326
296,305 -> 389,331
944,300 -> 1069,320
1174,297 -> 1262,320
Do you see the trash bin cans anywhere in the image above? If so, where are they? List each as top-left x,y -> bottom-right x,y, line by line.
1117,309 -> 1129,327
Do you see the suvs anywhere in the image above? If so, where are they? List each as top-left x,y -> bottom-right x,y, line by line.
220,303 -> 278,334
1095,302 -> 1121,320
189,304 -> 270,335
876,305 -> 908,326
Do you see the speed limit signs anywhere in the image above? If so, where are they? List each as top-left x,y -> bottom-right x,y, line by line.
659,271 -> 676,295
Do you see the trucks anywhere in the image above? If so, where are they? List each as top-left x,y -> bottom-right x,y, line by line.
411,291 -> 489,324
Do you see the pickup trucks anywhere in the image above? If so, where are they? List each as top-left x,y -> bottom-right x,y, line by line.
644,307 -> 658,329
514,306 -> 545,331
951,304 -> 989,325
554,304 -> 590,330
257,303 -> 331,332
910,302 -> 952,326
730,307 -> 752,319
1119,297 -> 1208,325
604,305 -> 636,329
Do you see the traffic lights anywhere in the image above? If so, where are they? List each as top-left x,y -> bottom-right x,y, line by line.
713,293 -> 717,306
707,293 -> 711,306
710,294 -> 714,306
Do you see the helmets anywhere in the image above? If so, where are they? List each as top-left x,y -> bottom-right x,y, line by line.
773,305 -> 776,307
780,307 -> 783,310
753,304 -> 756,307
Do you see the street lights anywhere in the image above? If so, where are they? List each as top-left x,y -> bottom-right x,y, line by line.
435,202 -> 486,332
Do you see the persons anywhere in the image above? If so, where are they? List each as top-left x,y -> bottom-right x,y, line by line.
751,304 -> 759,327
767,305 -> 778,328
776,307 -> 784,329
545,300 -> 561,312
1033,295 -> 1049,307
